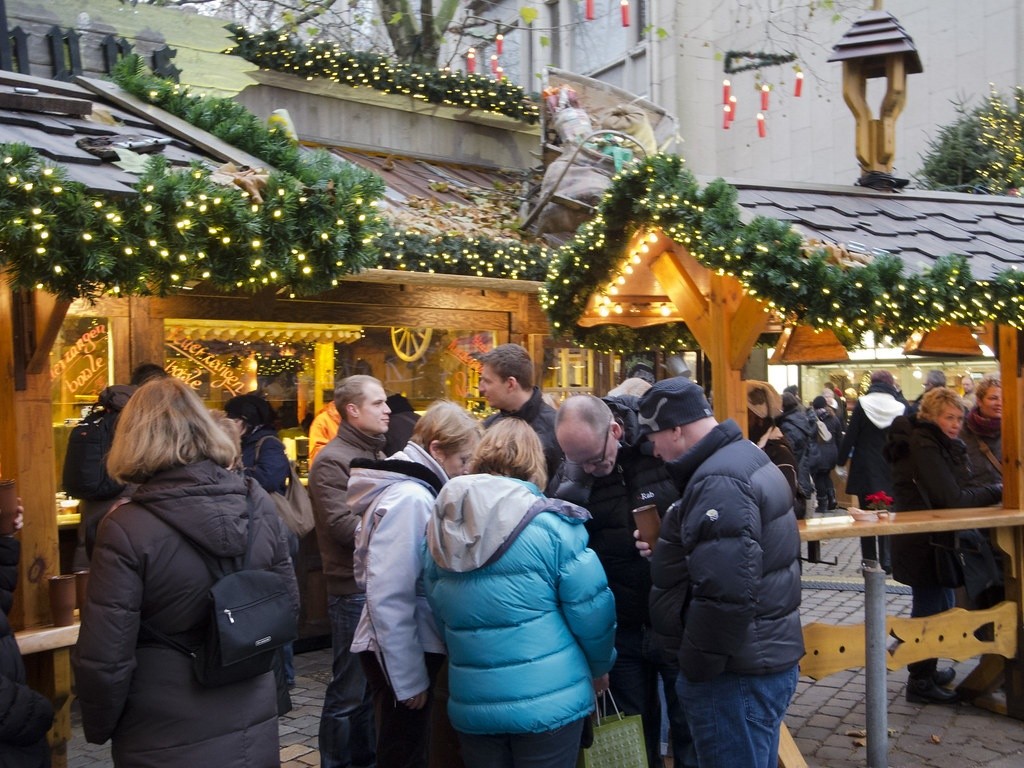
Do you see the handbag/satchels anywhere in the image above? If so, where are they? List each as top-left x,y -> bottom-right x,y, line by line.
254,435 -> 316,538
577,688 -> 649,768
815,416 -> 833,442
939,528 -> 1000,599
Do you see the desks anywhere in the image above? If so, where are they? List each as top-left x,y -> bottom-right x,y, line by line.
796,506 -> 1024,543
12,608 -> 80,654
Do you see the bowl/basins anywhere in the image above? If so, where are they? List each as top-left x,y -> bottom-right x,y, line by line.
851,511 -> 895,522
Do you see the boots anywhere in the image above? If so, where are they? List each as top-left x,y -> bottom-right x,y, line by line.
815,495 -> 829,512
826,488 -> 838,510
934,668 -> 956,685
906,676 -> 954,704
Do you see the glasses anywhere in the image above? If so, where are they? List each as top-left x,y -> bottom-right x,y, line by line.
921,382 -> 929,389
565,414 -> 612,466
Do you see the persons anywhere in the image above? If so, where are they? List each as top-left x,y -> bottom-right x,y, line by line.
882,374 -> 1002,705
223,396 -> 298,719
633,377 -> 807,768
837,370 -> 910,578
61,364 -> 298,768
476,343 -> 565,489
307,359 -> 372,468
346,401 -> 484,768
0,473 -> 55,768
746,379 -> 806,520
911,369 -> 969,418
776,382 -> 847,512
308,375 -> 420,768
422,418 -> 618,768
545,395 -> 681,768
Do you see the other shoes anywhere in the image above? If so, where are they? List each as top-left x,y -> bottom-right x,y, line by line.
883,566 -> 893,575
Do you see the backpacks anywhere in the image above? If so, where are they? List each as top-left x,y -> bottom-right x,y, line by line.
139,490 -> 302,691
764,442 -> 797,498
63,403 -> 128,502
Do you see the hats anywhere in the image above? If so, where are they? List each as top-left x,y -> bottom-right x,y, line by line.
224,394 -> 270,426
871,370 -> 894,385
385,393 -> 413,415
637,376 -> 714,435
833,387 -> 843,397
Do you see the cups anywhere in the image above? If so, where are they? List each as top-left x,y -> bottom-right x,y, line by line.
633,504 -> 661,557
0,479 -> 17,535
61,498 -> 80,515
49,569 -> 88,626
